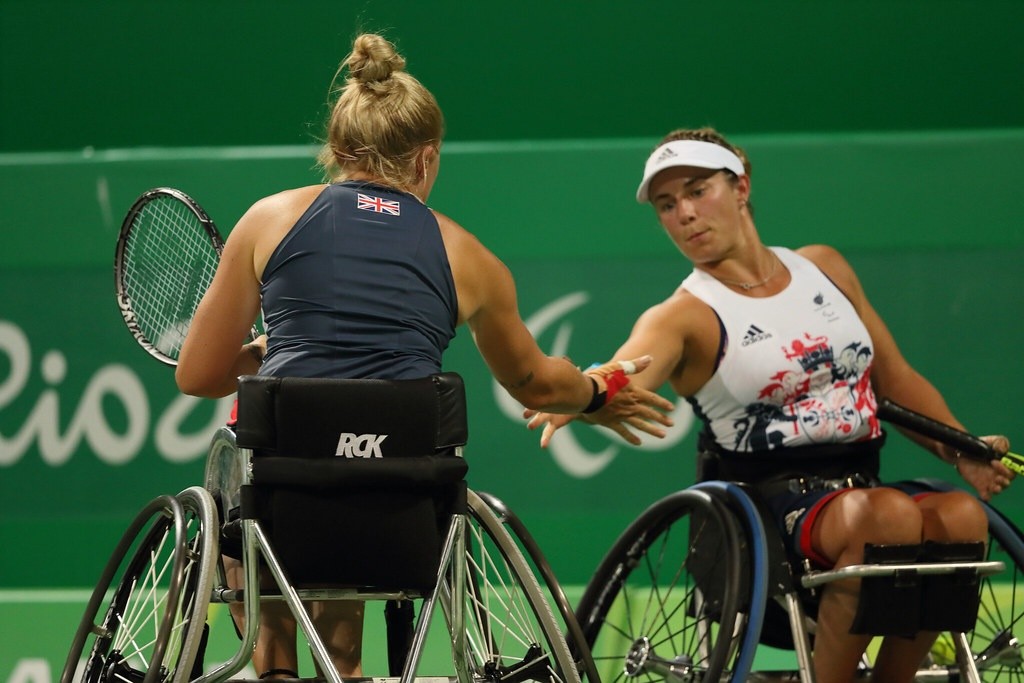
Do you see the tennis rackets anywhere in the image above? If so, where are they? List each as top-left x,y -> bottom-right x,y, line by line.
114,186 -> 266,368
874,396 -> 1024,475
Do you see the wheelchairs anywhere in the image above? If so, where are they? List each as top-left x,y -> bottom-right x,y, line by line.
555,427 -> 1024,683
59,372 -> 601,683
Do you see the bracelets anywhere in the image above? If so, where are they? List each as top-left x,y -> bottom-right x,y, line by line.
953,449 -> 962,469
579,372 -> 607,413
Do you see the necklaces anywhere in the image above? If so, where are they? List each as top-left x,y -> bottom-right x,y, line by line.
716,242 -> 778,290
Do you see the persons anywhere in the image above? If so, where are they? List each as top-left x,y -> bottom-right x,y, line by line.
174,31 -> 676,682
521,127 -> 1018,683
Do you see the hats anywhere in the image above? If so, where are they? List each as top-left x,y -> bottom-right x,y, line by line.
636,141 -> 746,203
